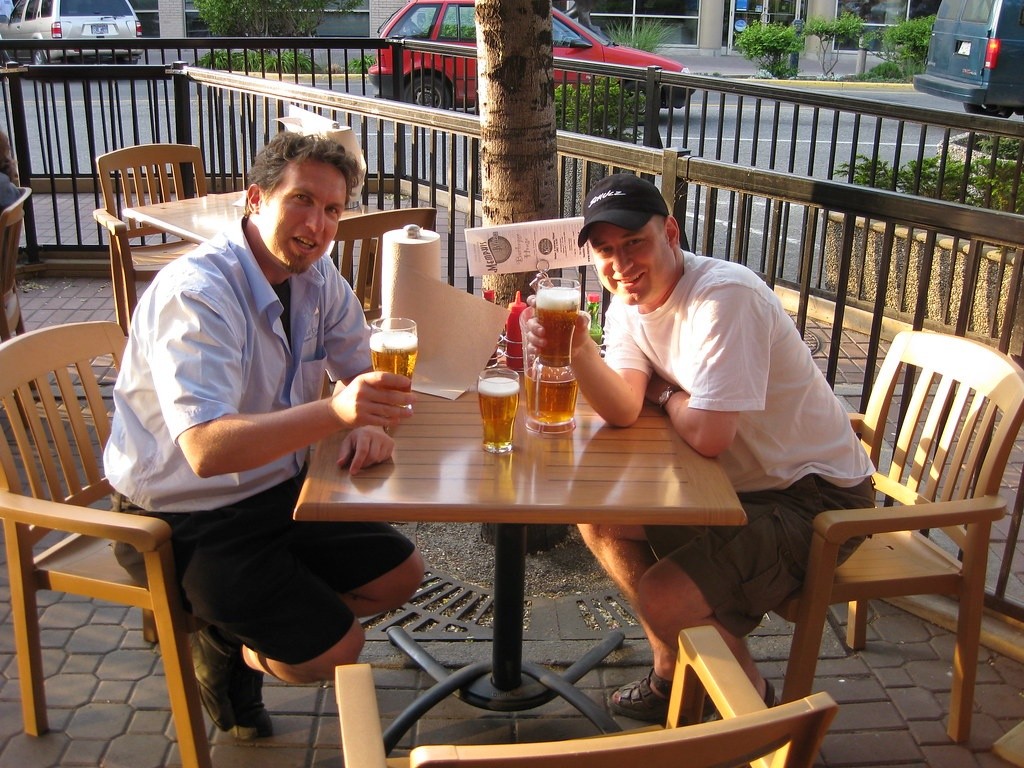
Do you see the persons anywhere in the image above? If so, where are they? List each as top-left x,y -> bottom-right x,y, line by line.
0,130 -> 21,211
526,172 -> 876,724
102,131 -> 424,739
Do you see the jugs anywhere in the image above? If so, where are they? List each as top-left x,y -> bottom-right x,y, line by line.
517,309 -> 591,431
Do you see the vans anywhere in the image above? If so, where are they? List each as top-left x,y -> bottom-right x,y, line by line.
913,0 -> 1024,118
0,0 -> 143,65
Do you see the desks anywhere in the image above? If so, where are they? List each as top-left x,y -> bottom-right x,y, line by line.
122,191 -> 359,246
292,357 -> 746,758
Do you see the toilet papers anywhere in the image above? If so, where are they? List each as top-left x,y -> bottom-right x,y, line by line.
381,222 -> 508,402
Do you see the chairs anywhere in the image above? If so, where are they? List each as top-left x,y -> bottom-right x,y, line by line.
92,144 -> 207,337
0,321 -> 212,768
333,206 -> 437,320
774,330 -> 1024,744
0,187 -> 37,430
334,627 -> 837,767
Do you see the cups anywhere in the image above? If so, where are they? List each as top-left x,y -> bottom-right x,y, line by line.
478,368 -> 520,454
369,317 -> 419,412
534,278 -> 582,368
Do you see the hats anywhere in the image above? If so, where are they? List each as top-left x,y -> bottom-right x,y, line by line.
578,174 -> 669,248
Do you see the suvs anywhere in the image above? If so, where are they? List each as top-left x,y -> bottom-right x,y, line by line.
368,0 -> 697,126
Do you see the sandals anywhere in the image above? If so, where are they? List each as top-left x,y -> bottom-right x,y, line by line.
608,667 -> 675,723
763,679 -> 776,708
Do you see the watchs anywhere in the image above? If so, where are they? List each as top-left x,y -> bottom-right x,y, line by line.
657,385 -> 684,409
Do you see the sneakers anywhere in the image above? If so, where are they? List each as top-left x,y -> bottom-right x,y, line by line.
189,625 -> 274,740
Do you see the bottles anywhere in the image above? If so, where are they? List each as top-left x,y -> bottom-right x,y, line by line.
506,290 -> 533,368
586,295 -> 603,344
480,290 -> 503,366
